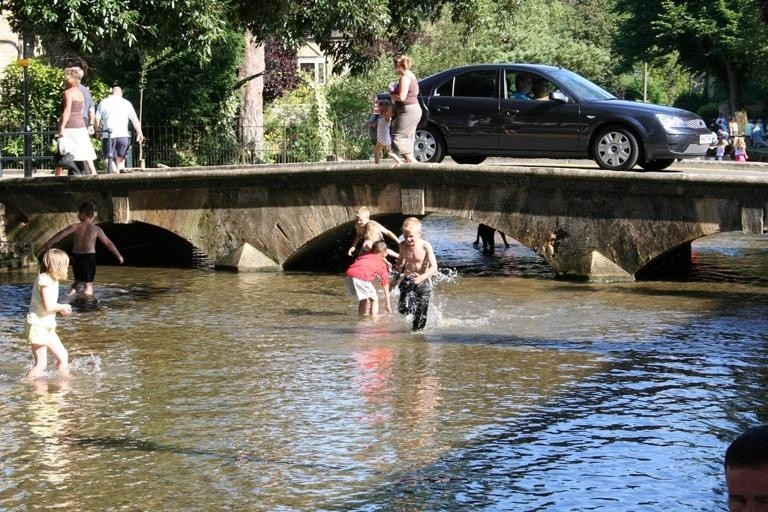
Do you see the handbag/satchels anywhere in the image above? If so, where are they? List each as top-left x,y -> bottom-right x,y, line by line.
341,223 -> 367,267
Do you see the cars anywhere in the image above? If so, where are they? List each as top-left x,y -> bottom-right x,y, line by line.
367,61 -> 715,172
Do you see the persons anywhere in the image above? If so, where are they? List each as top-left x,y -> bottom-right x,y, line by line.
21,247 -> 73,378
388,216 -> 437,332
94,85 -> 145,175
708,105 -> 768,162
388,54 -> 423,163
56,68 -> 99,175
54,59 -> 94,175
362,99 -> 403,163
345,240 -> 392,321
474,222 -> 511,250
348,205 -> 402,294
509,71 -> 535,100
35,201 -> 125,295
532,76 -> 550,100
